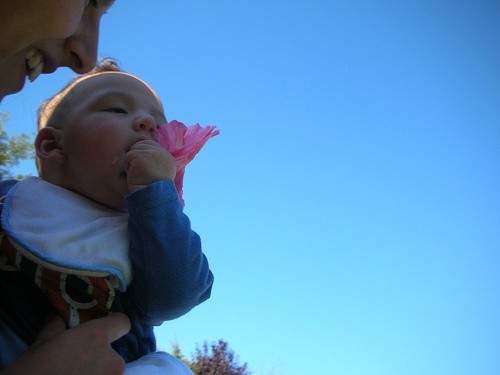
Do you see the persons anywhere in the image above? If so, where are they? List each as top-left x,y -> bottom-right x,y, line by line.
0,60 -> 214,374
1,2 -> 133,375
